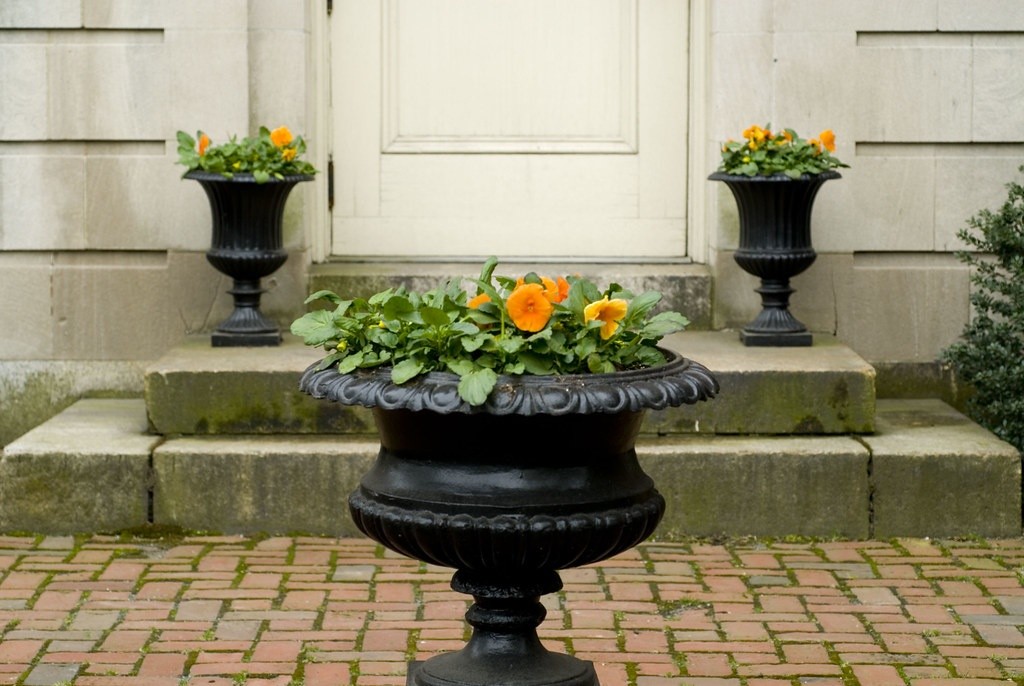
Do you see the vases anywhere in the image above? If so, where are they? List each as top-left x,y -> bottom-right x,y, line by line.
182,161 -> 315,349
707,169 -> 841,348
296,338 -> 720,686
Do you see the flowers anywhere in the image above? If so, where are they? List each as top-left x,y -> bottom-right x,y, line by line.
716,124 -> 844,173
287,255 -> 690,406
177,121 -> 307,178
